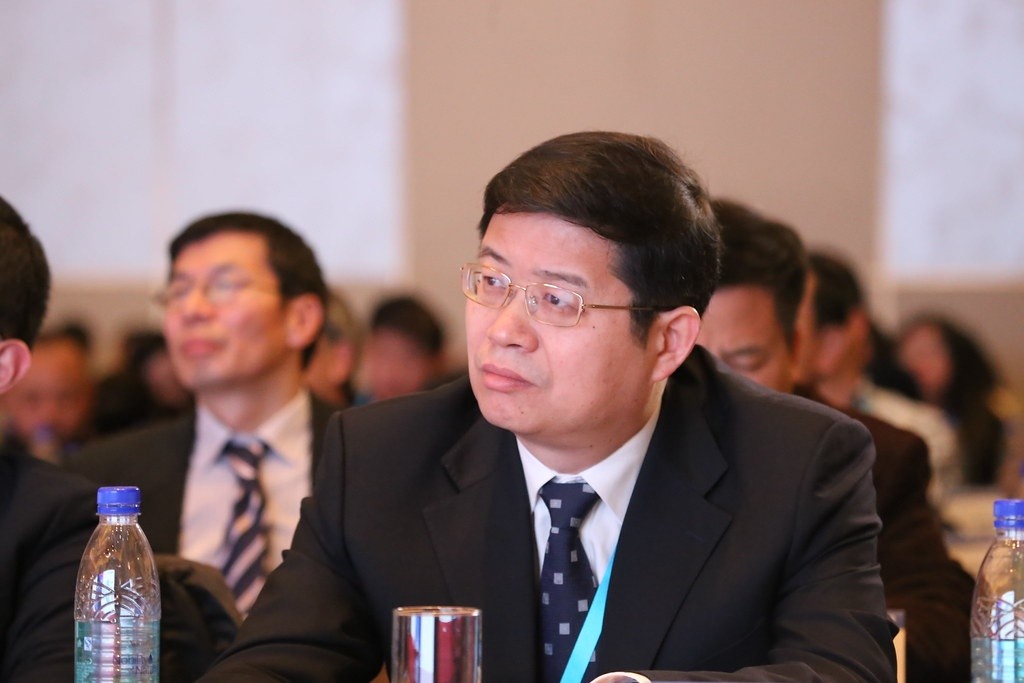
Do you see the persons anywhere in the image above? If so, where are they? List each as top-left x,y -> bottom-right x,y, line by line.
196,131 -> 899,683
0,199 -> 1011,683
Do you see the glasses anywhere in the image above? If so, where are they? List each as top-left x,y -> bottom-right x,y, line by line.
461,260 -> 666,330
151,269 -> 294,306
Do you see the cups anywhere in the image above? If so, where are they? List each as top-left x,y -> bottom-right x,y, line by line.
388,605 -> 483,683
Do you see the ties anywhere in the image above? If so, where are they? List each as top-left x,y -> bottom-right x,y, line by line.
216,438 -> 274,623
534,478 -> 600,683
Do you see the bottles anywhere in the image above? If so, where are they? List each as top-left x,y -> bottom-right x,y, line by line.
72,485 -> 163,683
967,497 -> 1024,683
